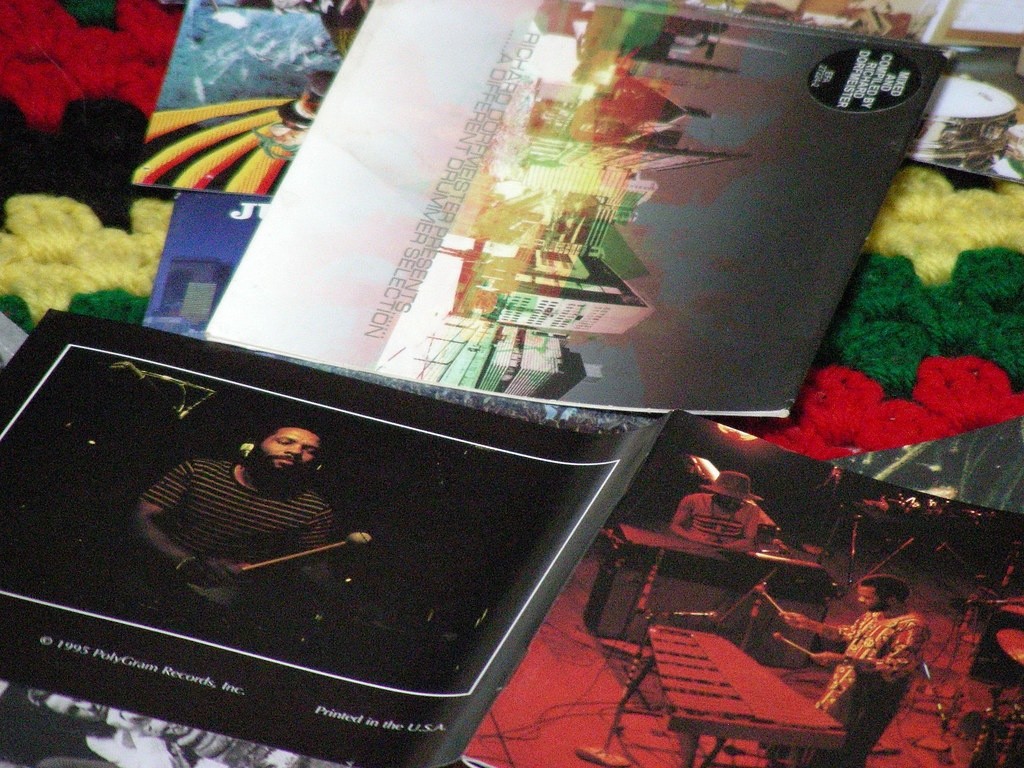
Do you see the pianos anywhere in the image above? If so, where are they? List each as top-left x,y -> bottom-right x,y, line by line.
581,521 -> 835,637
861,498 -> 1002,531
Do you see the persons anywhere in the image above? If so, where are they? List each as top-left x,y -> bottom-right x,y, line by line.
110,419 -> 375,653
777,572 -> 930,768
670,466 -> 781,556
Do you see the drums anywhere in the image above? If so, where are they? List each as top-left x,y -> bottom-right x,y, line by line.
906,72 -> 1021,174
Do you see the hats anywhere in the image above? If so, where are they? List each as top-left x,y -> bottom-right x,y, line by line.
699,470 -> 765,502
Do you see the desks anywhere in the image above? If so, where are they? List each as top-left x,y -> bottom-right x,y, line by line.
577,528 -> 830,669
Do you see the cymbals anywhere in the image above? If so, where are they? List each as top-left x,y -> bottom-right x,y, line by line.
994,627 -> 1024,666
